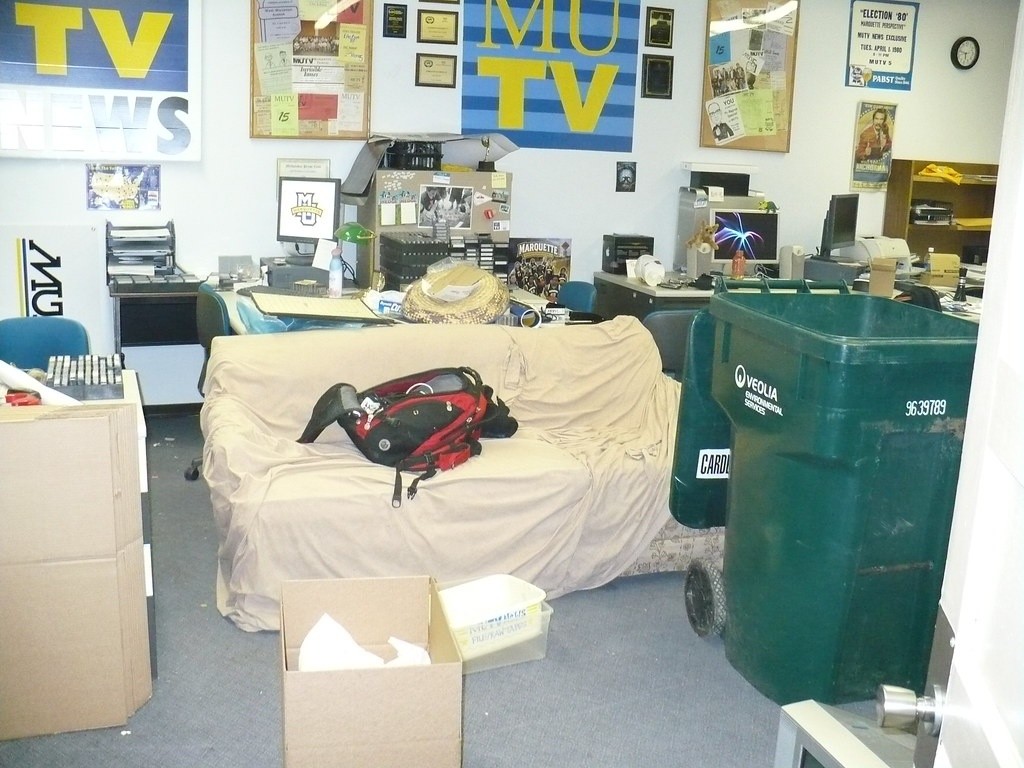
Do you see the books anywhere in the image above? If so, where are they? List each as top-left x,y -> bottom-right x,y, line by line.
47,354 -> 123,384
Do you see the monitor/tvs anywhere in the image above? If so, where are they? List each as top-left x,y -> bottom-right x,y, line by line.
277,176 -> 341,265
709,209 -> 780,278
810,193 -> 859,262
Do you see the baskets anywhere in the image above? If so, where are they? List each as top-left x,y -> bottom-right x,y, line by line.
380,138 -> 441,171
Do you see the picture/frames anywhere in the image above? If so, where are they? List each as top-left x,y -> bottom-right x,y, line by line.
644,6 -> 675,48
641,53 -> 674,100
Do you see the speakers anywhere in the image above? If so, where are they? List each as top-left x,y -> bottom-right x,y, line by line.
687,243 -> 711,279
779,245 -> 805,280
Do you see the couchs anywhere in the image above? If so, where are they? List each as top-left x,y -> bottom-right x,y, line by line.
198,314 -> 727,635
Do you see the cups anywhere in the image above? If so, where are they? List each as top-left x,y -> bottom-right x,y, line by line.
869,257 -> 897,298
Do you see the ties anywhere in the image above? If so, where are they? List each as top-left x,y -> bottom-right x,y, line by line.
877,129 -> 880,145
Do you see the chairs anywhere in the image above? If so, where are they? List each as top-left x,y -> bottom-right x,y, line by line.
0,316 -> 88,372
556,280 -> 598,317
184,282 -> 230,481
643,309 -> 700,383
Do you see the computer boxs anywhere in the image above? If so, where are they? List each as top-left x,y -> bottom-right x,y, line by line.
260,257 -> 331,288
805,258 -> 869,286
673,186 -> 766,272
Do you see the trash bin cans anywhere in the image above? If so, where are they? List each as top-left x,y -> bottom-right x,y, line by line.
667,277 -> 979,707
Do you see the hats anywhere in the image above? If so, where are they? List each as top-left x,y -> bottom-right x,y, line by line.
402,264 -> 509,324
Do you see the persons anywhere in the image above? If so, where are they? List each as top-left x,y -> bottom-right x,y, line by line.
507,256 -> 568,299
711,63 -> 746,95
419,187 -> 471,224
293,31 -> 339,56
856,109 -> 892,163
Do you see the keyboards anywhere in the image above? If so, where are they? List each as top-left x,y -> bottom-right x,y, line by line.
237,286 -> 323,298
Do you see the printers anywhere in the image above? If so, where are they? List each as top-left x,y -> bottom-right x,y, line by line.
840,234 -> 925,281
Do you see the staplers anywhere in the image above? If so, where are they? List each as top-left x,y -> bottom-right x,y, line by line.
565,310 -> 607,325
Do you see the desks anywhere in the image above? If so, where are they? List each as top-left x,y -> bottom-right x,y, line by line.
214,287 -> 390,336
592,263 -> 988,324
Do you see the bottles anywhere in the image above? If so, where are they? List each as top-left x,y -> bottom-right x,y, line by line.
923,247 -> 935,273
329,248 -> 343,297
732,250 -> 745,280
953,267 -> 968,302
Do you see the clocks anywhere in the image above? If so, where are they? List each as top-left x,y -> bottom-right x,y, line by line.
951,36 -> 980,71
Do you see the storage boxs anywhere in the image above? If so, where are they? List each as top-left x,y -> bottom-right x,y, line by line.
0,401 -> 153,742
921,253 -> 961,288
277,576 -> 464,768
453,600 -> 554,675
437,574 -> 547,663
386,141 -> 444,171
601,233 -> 654,275
867,257 -> 901,298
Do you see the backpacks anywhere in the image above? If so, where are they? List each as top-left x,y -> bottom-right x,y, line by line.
297,365 -> 518,471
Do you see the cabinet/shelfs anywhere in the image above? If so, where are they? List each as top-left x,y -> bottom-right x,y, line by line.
105,220 -> 177,279
882,159 -> 999,266
